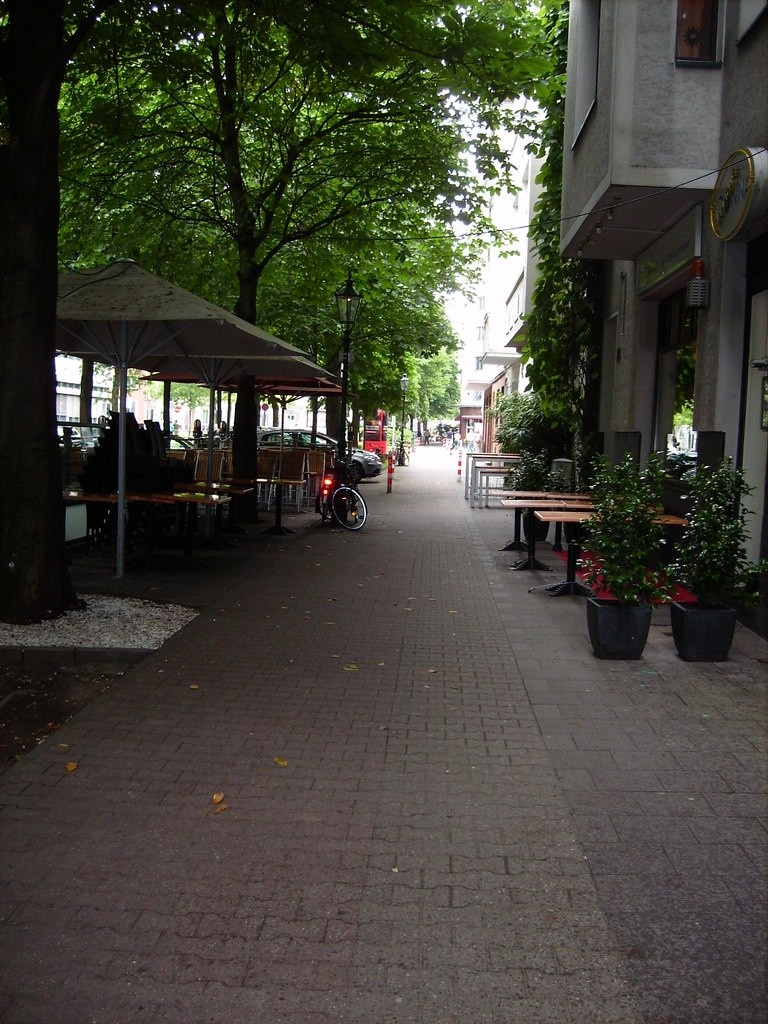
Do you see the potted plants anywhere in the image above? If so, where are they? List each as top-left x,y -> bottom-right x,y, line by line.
503,447 -> 768,663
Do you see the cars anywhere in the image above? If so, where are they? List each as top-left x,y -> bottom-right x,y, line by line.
256,429 -> 382,484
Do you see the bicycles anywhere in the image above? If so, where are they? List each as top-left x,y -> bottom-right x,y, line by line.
315,449 -> 368,532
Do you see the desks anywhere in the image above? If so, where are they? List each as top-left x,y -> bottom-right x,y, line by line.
534,511 -> 688,598
61,484 -> 254,571
500,499 -> 664,572
465,453 -> 523,508
487,489 -> 624,551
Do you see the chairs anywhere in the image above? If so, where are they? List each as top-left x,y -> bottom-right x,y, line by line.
165,447 -> 335,514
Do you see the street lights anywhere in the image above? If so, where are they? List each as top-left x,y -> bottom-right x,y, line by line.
331,273 -> 363,526
398,373 -> 409,466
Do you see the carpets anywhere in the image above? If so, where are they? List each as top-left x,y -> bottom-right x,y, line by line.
548,547 -> 698,603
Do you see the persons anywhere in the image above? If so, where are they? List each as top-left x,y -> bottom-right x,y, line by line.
169,420 -> 181,436
423,429 -> 430,445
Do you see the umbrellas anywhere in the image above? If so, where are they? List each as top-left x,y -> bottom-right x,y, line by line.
57,257 -> 311,576
139,356 -> 357,536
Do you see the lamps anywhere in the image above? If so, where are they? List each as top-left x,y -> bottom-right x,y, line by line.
688,257 -> 709,310
